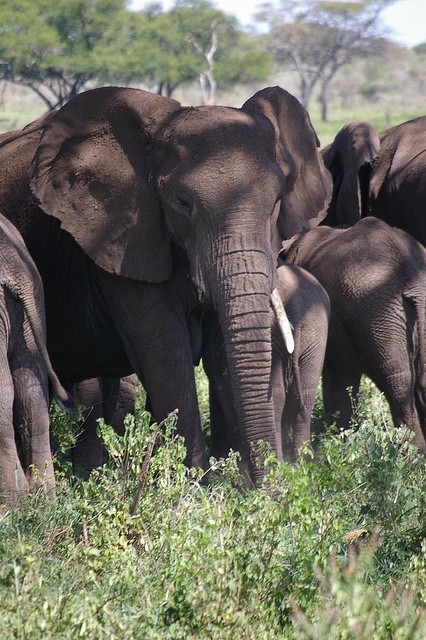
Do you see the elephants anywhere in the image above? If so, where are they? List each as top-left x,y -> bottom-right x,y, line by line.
0,88 -> 426,513
